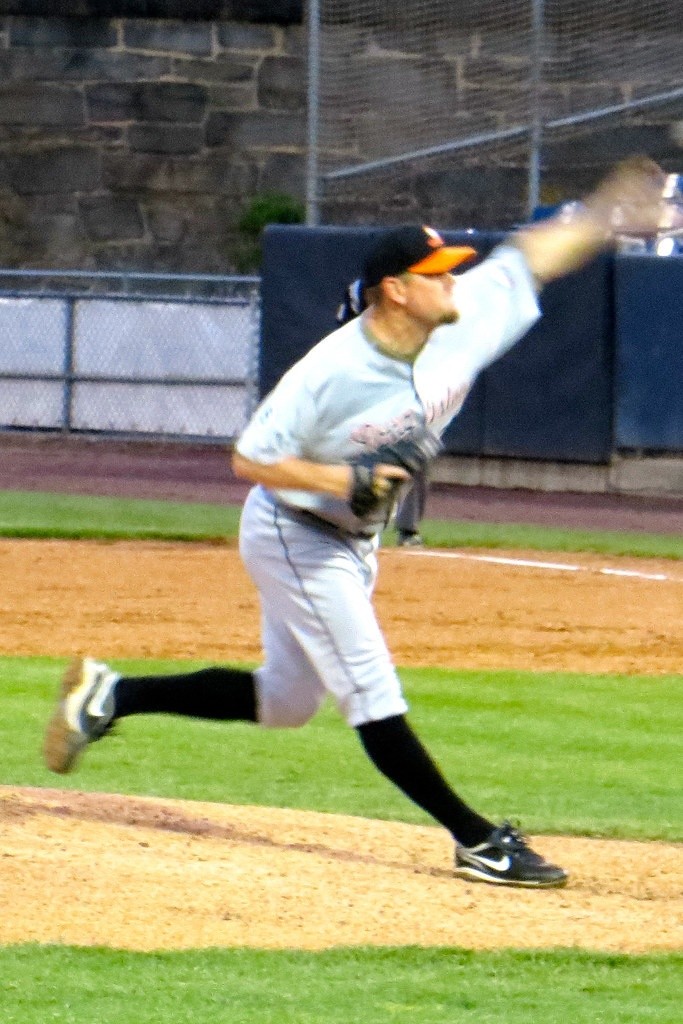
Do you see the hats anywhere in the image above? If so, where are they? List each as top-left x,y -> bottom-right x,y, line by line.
361,222 -> 476,287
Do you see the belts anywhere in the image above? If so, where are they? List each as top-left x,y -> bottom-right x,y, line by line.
298,509 -> 375,540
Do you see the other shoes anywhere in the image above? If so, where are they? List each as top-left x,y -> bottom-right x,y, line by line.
397,531 -> 421,547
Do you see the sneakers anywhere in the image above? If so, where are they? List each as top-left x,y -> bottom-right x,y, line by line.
455,816 -> 568,889
46,657 -> 122,774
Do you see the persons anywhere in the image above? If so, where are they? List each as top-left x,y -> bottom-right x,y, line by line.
42,162 -> 675,889
